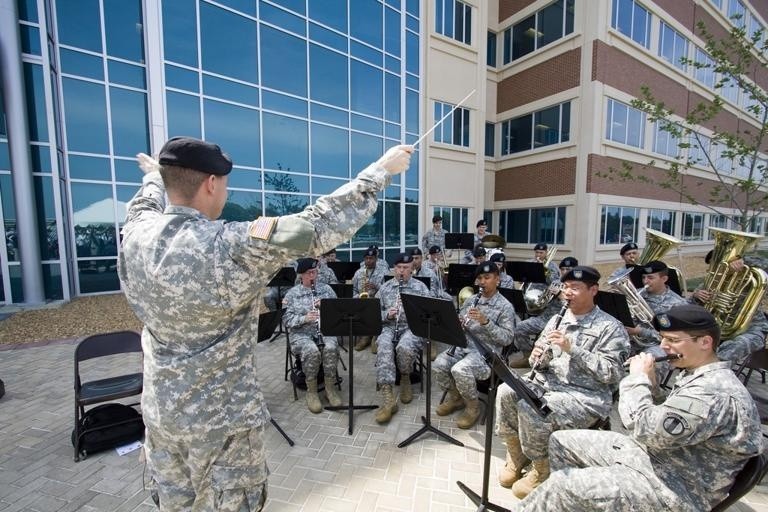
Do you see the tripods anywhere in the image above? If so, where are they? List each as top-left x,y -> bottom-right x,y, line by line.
398,317 -> 464,447
269,285 -> 290,342
457,368 -> 510,512
324,318 -> 379,435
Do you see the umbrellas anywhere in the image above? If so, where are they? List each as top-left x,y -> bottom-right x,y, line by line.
72,198 -> 127,229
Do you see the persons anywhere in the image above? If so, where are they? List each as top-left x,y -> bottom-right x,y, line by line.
115,136 -> 415,512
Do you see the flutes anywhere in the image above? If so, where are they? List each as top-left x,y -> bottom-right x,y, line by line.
621,353 -> 684,370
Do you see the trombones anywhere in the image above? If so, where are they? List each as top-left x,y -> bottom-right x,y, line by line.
518,245 -> 558,290
435,257 -> 443,296
438,246 -> 449,273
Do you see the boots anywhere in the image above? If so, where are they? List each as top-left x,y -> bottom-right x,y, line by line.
355,335 -> 371,352
512,454 -> 550,500
305,378 -> 322,413
400,374 -> 413,404
497,435 -> 531,487
510,350 -> 531,369
371,335 -> 377,354
436,387 -> 465,416
458,392 -> 482,429
376,383 -> 399,424
430,339 -> 438,360
324,375 -> 342,407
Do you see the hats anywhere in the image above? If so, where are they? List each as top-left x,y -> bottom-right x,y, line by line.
474,248 -> 486,257
642,260 -> 667,275
431,216 -> 443,224
363,244 -> 379,257
652,305 -> 719,332
476,260 -> 500,275
428,245 -> 441,254
560,256 -> 578,269
327,248 -> 336,254
477,220 -> 487,228
621,242 -> 637,255
560,264 -> 600,287
158,136 -> 232,175
413,249 -> 422,255
533,243 -> 548,251
395,253 -> 413,265
490,252 -> 506,262
297,257 -> 318,274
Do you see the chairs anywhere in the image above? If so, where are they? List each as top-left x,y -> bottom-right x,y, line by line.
517,263 -> 767,512
282,263 -> 517,426
71,329 -> 149,463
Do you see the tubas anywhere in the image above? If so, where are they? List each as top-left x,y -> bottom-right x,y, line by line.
698,225 -> 768,341
605,266 -> 667,346
636,228 -> 686,293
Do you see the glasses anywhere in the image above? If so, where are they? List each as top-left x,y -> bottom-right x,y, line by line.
657,334 -> 698,344
561,285 -> 587,291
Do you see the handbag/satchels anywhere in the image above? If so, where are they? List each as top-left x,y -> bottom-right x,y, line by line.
71,402 -> 144,460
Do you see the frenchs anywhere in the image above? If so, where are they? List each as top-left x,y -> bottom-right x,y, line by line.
522,279 -> 565,316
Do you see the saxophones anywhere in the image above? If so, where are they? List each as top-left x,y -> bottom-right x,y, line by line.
359,265 -> 370,299
